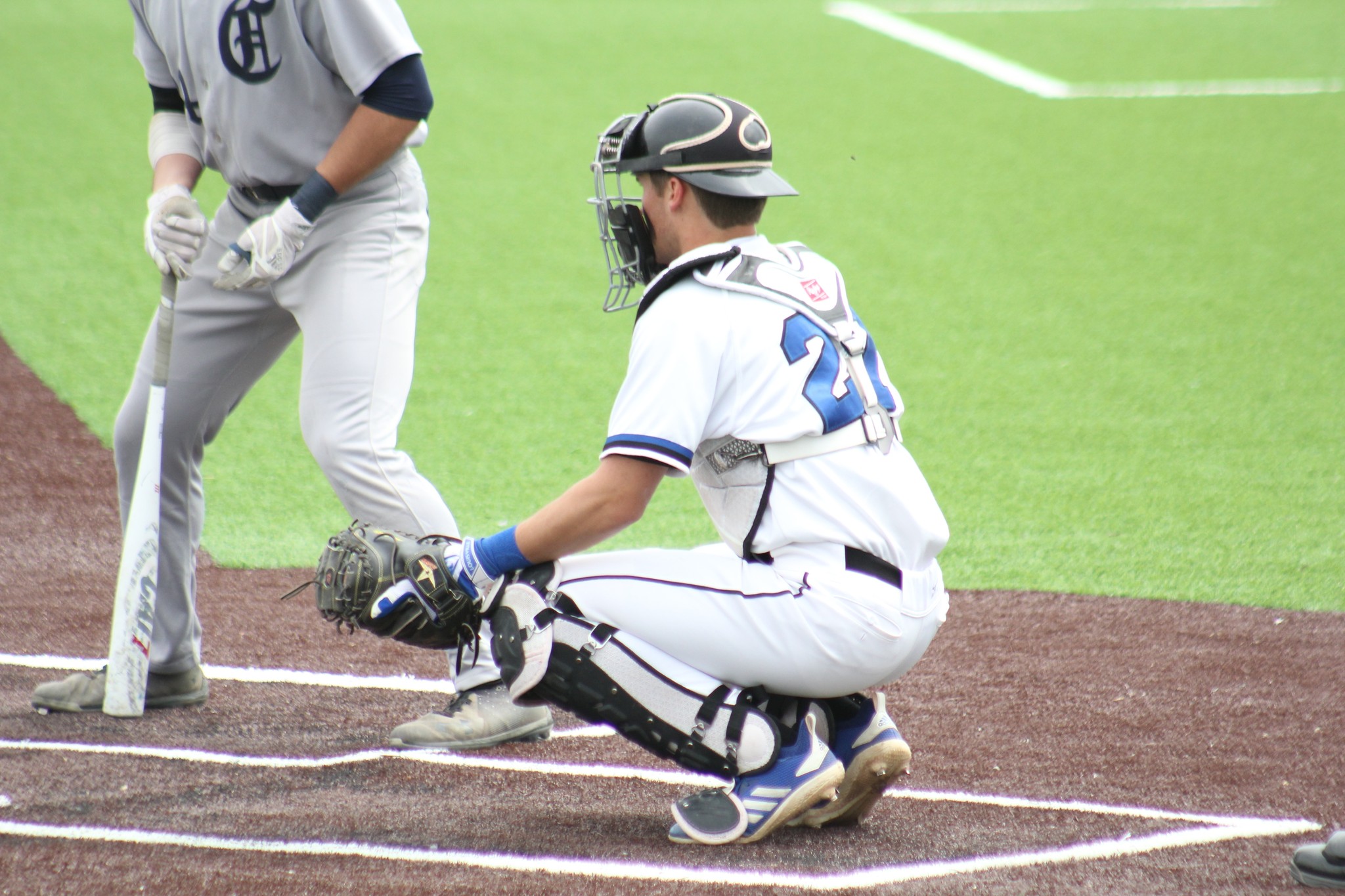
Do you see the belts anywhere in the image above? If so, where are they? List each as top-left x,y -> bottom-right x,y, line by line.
233,182 -> 305,203
844,546 -> 903,590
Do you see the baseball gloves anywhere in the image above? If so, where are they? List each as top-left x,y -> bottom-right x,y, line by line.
312,523 -> 482,654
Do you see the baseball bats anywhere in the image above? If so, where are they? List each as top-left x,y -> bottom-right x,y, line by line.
100,219 -> 189,720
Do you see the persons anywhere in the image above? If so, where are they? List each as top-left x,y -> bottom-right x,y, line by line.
277,93 -> 950,842
32,0 -> 555,748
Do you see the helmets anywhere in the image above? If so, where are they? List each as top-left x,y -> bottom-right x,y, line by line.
627,94 -> 799,196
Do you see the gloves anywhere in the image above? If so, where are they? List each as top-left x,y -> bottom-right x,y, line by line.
145,184 -> 207,282
213,196 -> 319,291
370,535 -> 502,622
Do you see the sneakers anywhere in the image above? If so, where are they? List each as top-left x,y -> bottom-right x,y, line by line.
1288,831 -> 1345,890
31,662 -> 210,712
669,712 -> 845,844
785,691 -> 911,828
390,683 -> 554,750
